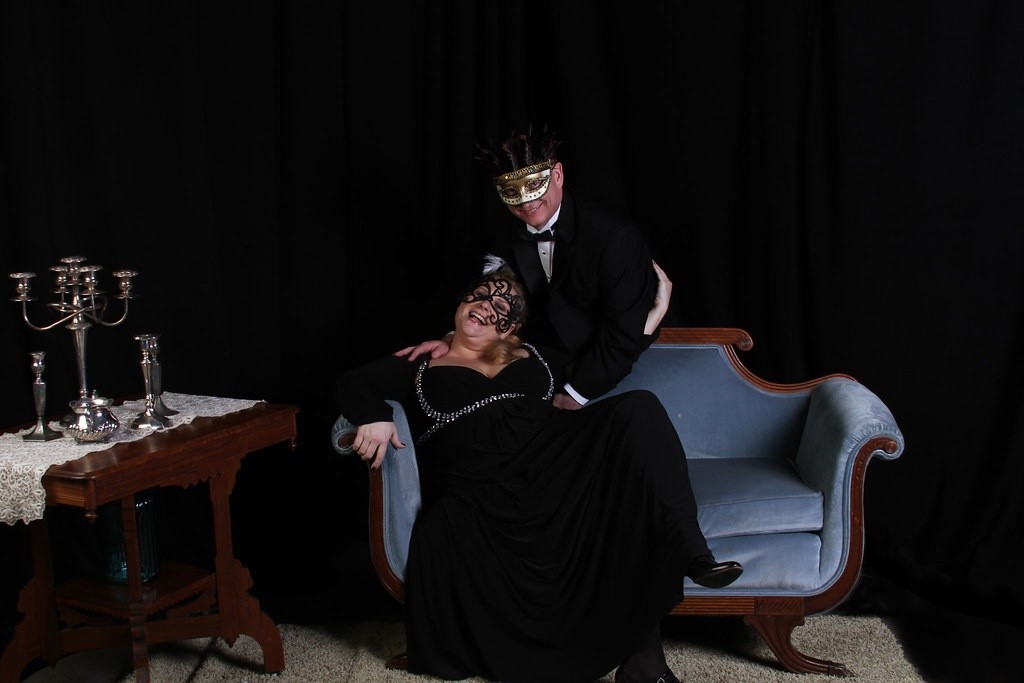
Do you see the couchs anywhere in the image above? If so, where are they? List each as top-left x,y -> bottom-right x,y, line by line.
331,324 -> 907,678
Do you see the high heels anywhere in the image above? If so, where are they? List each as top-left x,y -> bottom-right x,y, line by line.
648,553 -> 743,612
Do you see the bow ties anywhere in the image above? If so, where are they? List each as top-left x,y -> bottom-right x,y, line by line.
519,230 -> 555,242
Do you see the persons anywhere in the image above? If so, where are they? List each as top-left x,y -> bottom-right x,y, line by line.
339,266 -> 741,683
399,114 -> 728,421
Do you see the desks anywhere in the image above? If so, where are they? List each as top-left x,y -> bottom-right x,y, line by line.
0,392 -> 301,683
